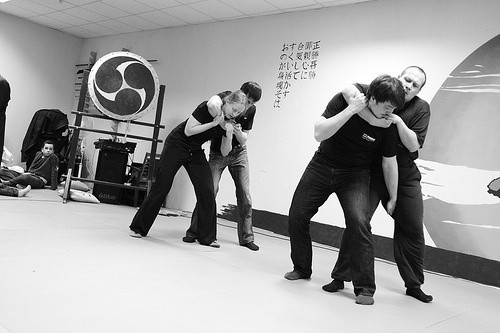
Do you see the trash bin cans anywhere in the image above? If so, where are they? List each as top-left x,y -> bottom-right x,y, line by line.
91,138 -> 137,205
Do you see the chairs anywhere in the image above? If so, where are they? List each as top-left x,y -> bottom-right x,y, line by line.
125,153 -> 160,186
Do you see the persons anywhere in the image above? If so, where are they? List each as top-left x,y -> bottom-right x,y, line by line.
130,90 -> 248,247
1,141 -> 59,191
322,64 -> 434,303
182,81 -> 260,250
0,183 -> 32,197
0,75 -> 11,165
284,76 -> 405,305
